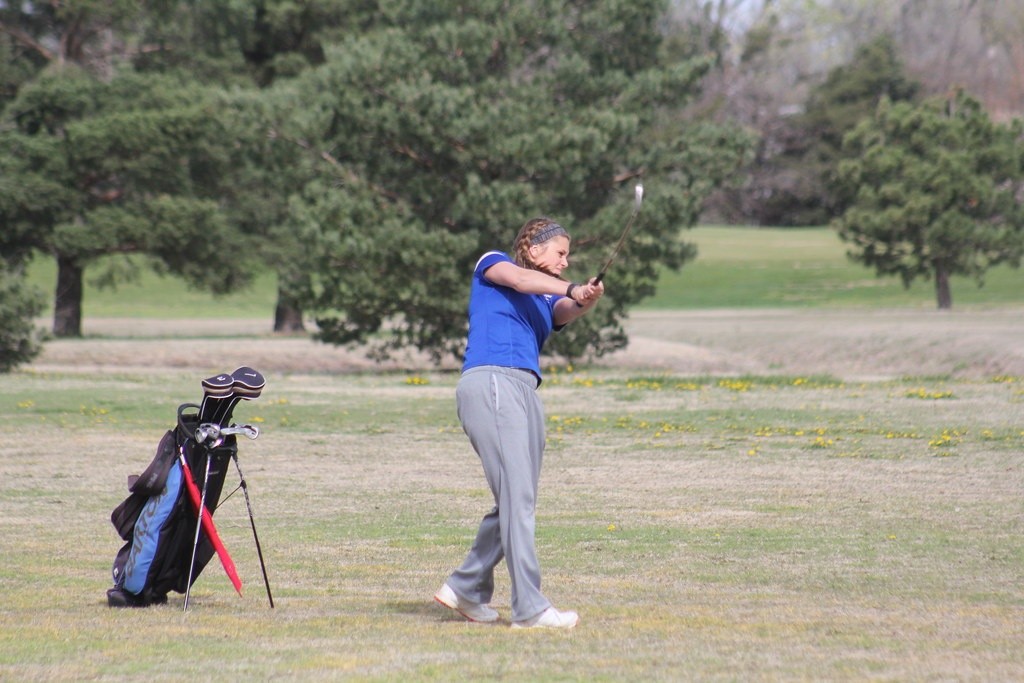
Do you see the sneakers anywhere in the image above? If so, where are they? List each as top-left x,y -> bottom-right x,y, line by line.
433,583 -> 499,624
511,605 -> 578,630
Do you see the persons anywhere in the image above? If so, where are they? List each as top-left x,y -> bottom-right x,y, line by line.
433,218 -> 605,630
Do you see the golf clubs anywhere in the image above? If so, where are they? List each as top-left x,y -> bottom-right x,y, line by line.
195,423 -> 260,452
576,183 -> 643,308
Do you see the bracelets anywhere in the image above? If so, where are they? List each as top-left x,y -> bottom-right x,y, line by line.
576,302 -> 583,308
566,284 -> 577,300
